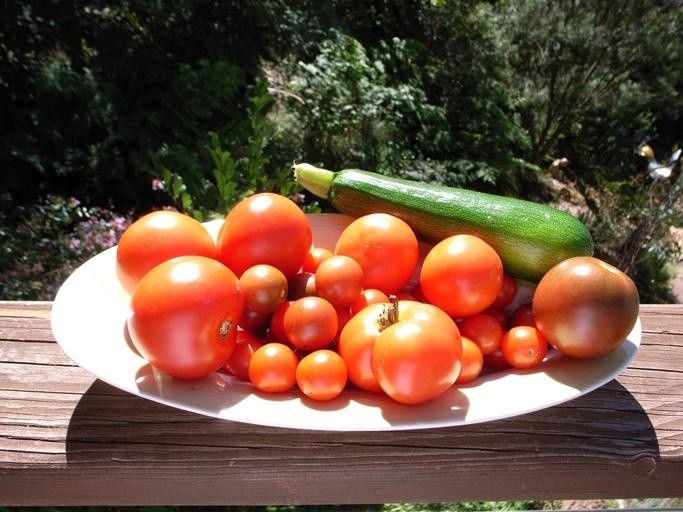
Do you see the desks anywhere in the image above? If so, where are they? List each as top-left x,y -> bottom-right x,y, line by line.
2,299 -> 682,505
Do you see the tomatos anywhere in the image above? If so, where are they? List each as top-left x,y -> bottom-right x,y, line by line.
116,191 -> 639,404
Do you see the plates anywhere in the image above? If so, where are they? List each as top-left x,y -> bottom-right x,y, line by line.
48,208 -> 647,437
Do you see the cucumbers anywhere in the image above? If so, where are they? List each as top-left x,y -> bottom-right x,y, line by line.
293,159 -> 594,288
